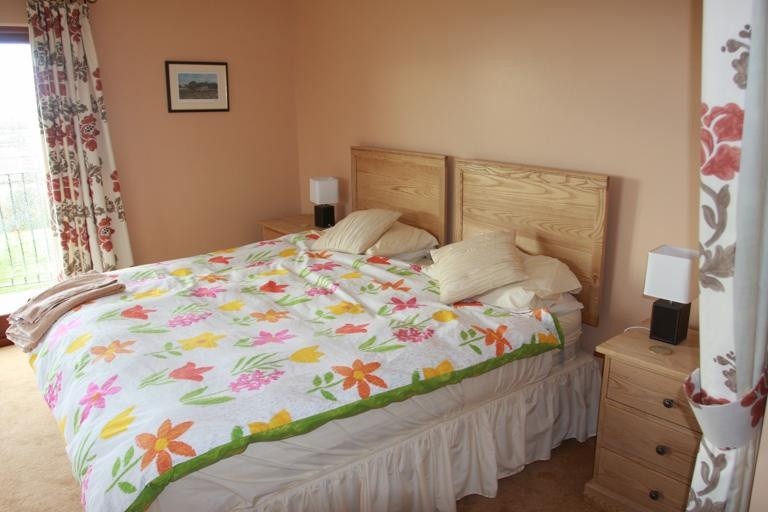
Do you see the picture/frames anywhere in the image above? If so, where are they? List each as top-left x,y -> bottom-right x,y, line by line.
164,59 -> 230,112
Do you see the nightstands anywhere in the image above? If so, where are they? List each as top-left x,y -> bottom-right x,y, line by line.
257,214 -> 314,241
584,319 -> 704,512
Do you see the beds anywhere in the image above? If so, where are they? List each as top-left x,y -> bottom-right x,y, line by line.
26,227 -> 602,511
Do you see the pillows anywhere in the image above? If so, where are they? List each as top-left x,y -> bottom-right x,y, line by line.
314,209 -> 582,313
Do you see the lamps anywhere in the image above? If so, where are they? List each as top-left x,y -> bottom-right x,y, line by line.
642,242 -> 700,345
307,176 -> 340,229
